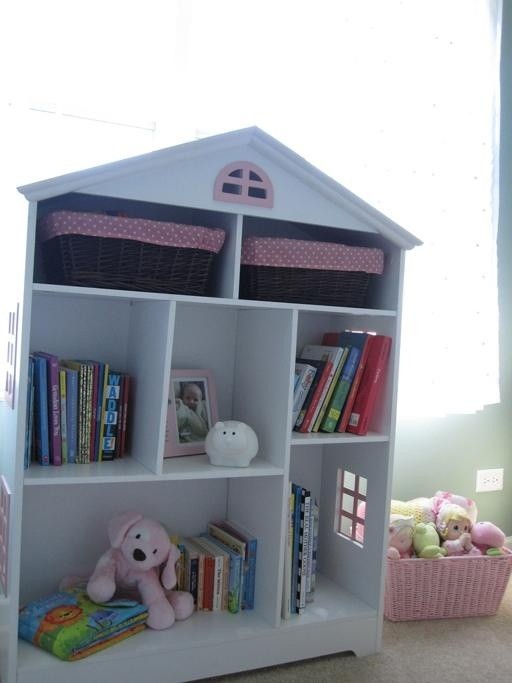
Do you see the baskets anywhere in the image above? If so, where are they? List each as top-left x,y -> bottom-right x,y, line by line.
350,524 -> 512,622
37,210 -> 226,296
240,236 -> 385,307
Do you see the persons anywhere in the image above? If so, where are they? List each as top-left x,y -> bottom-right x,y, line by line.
175,382 -> 210,444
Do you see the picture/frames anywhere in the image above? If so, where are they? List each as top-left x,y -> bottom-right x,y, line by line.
161,367 -> 221,459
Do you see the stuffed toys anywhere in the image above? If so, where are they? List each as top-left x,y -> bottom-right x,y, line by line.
87,511 -> 194,631
354,489 -> 506,560
204,420 -> 259,468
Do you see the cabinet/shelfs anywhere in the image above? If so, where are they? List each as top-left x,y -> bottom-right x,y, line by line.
0,111 -> 425,682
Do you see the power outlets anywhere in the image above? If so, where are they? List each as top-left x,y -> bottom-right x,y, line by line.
475,464 -> 505,494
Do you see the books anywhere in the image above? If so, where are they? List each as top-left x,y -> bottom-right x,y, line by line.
18,577 -> 148,661
169,519 -> 258,614
291,331 -> 392,436
281,481 -> 330,621
24,351 -> 130,471
